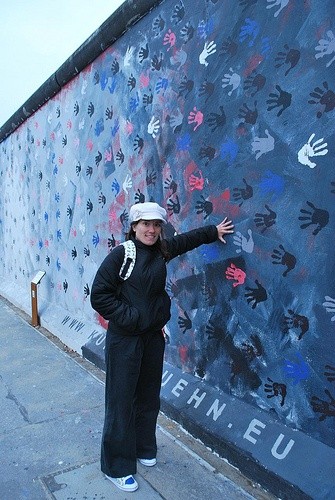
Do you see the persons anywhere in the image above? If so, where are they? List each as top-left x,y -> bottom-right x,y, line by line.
90,202 -> 233,491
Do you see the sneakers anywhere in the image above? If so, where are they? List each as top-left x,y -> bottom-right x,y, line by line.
104,473 -> 139,491
138,458 -> 157,467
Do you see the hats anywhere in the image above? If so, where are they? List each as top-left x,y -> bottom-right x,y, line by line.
129,202 -> 168,225
